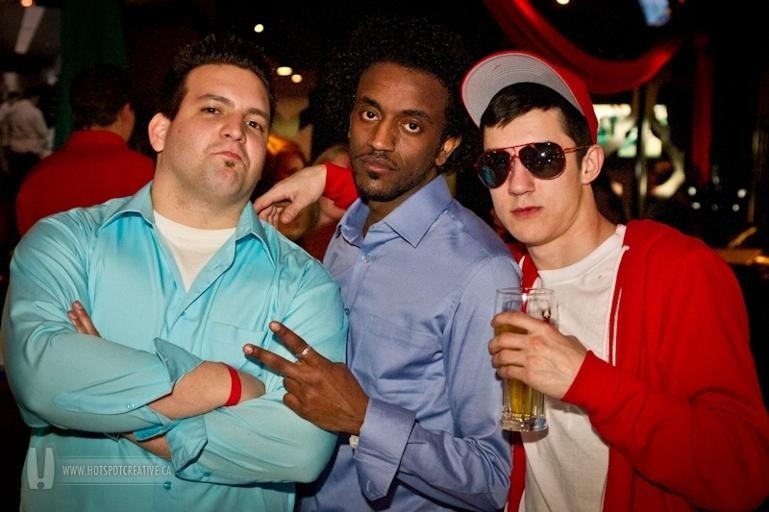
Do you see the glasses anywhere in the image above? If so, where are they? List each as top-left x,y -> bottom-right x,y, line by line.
474,141 -> 590,189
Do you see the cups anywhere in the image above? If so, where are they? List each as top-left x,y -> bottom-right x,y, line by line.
496,286 -> 559,433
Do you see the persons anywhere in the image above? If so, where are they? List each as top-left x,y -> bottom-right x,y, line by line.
252,53 -> 769,512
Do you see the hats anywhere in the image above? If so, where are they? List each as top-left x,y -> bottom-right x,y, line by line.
460,51 -> 599,145
